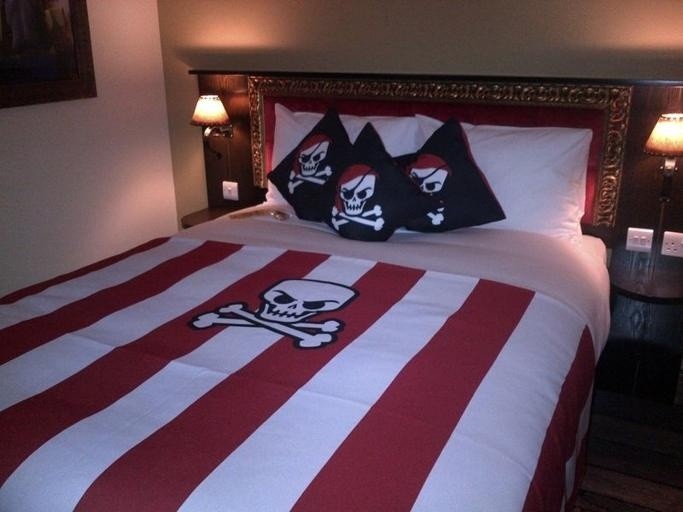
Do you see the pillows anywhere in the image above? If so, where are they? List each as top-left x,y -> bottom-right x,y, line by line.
266,100 -> 423,208
412,110 -> 593,247
392,113 -> 505,233
268,103 -> 353,221
321,123 -> 443,243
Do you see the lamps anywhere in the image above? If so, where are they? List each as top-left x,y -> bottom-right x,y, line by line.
641,112 -> 682,206
189,94 -> 234,157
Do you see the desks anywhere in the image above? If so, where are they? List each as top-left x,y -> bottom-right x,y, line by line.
596,256 -> 683,402
177,205 -> 244,231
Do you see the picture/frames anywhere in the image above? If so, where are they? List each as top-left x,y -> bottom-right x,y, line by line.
0,1 -> 97,111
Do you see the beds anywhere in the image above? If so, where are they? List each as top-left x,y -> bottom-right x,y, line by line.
0,66 -> 632,511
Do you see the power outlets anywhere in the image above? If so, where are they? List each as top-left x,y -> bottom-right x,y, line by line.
660,230 -> 682,259
